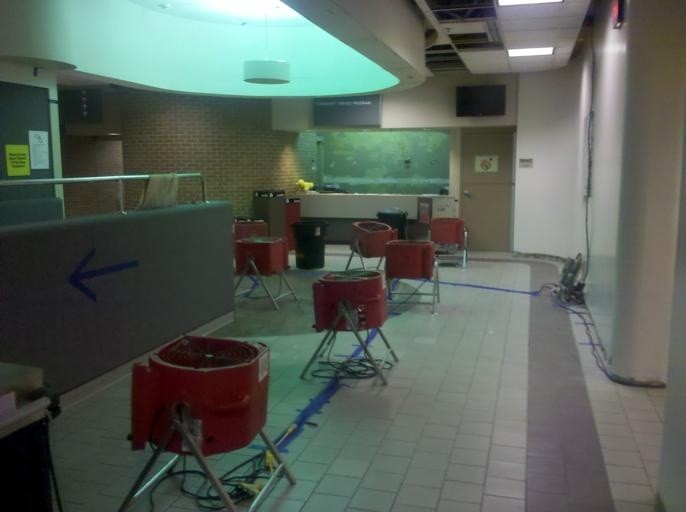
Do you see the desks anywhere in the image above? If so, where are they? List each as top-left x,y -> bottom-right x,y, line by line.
0,390 -> 62,512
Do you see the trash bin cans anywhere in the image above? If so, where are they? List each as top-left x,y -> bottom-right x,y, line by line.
0,396 -> 54,512
377,208 -> 408,241
294,219 -> 327,269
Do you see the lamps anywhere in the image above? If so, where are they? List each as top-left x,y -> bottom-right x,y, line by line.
243,60 -> 290,84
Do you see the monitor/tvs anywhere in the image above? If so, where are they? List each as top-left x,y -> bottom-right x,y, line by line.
455,84 -> 506,118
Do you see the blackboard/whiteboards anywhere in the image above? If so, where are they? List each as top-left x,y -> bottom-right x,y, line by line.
319,127 -> 450,195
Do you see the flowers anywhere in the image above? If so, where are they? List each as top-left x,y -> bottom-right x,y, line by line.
296,179 -> 313,192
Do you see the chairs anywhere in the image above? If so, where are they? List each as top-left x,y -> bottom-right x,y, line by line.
0,362 -> 62,512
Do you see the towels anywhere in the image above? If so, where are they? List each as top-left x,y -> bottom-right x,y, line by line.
134,173 -> 179,208
